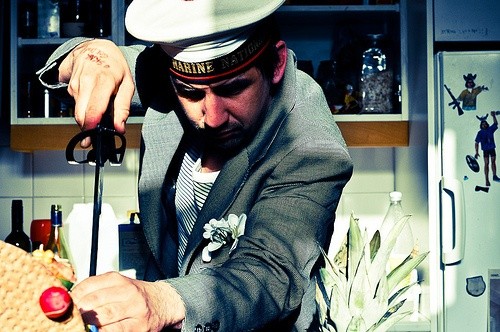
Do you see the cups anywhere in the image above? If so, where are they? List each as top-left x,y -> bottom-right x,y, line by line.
30,220 -> 52,251
21,81 -> 40,116
19,2 -> 37,37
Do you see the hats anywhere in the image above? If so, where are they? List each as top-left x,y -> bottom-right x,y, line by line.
125,0 -> 287,80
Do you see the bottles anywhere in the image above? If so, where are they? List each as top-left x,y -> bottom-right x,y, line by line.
96,1 -> 105,36
360,34 -> 393,112
379,192 -> 418,322
63,0 -> 85,36
46,204 -> 76,275
4,199 -> 32,252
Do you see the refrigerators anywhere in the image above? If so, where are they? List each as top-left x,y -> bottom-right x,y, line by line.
427,0 -> 500,332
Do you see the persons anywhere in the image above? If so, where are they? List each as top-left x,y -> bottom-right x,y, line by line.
457,79 -> 482,110
474,111 -> 500,186
36,0 -> 353,332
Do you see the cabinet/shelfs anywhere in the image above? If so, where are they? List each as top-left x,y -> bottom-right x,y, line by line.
0,0 -> 410,153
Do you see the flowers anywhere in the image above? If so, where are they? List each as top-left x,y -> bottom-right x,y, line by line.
201,214 -> 248,262
